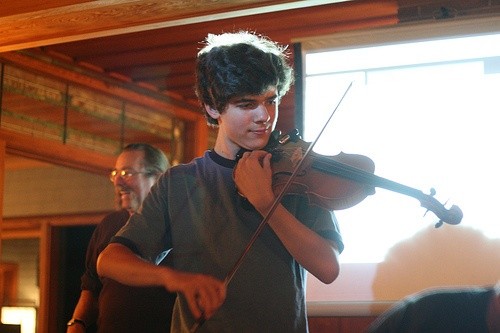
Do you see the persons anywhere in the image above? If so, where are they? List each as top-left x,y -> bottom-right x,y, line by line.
66,143 -> 176,333
96,30 -> 344,333
364,280 -> 500,333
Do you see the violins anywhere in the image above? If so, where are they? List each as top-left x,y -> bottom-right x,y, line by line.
236,129 -> 464,228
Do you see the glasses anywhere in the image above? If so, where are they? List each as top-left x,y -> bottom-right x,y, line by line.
110,169 -> 156,182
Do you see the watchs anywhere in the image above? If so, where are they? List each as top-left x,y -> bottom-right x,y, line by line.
67,318 -> 88,330
